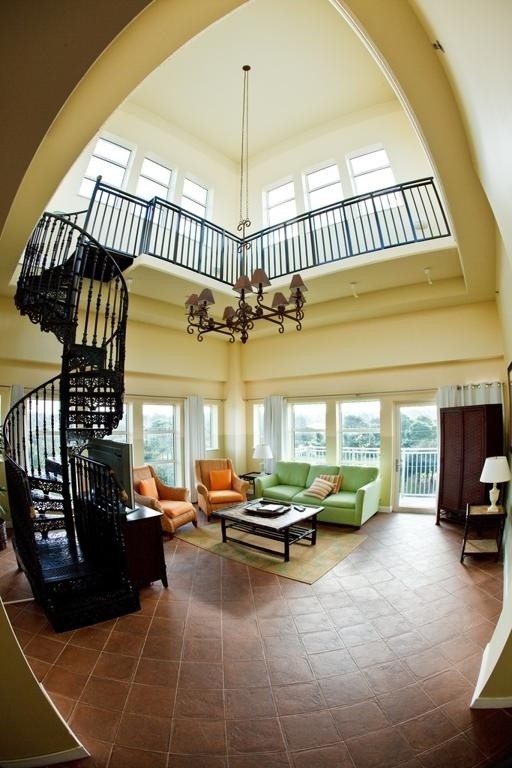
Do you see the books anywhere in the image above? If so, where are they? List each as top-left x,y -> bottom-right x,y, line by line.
257,503 -> 284,513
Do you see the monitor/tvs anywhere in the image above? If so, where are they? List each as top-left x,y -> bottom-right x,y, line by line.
87,438 -> 134,510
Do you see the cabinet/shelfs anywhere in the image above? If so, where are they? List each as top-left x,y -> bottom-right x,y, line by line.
460,503 -> 506,565
436,404 -> 502,537
73,491 -> 168,598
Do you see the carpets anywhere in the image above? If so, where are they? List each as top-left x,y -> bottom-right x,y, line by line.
174,522 -> 369,585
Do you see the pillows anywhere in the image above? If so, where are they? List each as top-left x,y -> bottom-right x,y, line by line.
304,476 -> 344,501
140,478 -> 159,501
209,470 -> 232,490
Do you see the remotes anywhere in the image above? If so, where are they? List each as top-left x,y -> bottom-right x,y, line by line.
294,507 -> 303,512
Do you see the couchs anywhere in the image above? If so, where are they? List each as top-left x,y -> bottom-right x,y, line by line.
194,458 -> 250,523
255,460 -> 382,530
124,464 -> 197,540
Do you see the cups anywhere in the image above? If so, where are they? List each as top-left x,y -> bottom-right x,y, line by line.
260,463 -> 265,475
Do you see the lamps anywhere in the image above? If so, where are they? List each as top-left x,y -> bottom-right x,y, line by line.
479,456 -> 512,513
253,445 -> 273,477
185,64 -> 308,343
